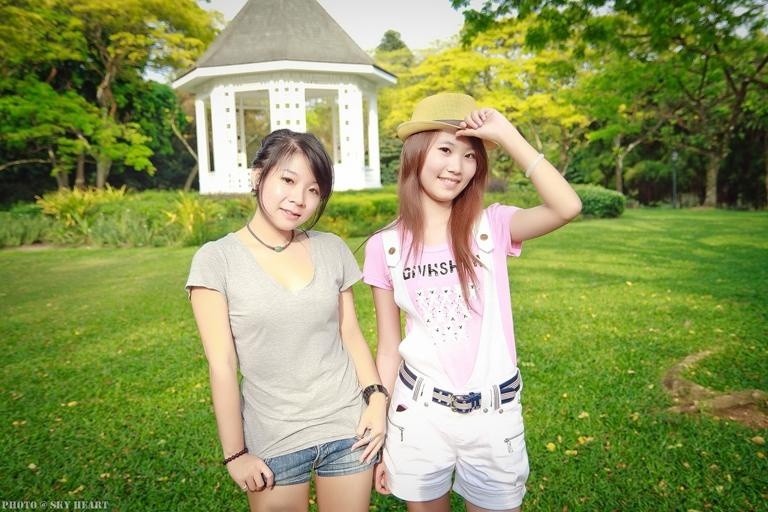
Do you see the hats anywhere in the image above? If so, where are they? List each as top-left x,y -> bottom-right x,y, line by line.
396,88 -> 500,152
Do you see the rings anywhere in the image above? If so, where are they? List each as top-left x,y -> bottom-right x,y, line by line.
241,485 -> 247,490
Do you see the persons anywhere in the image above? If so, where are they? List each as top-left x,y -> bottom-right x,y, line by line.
361,92 -> 585,510
181,127 -> 391,511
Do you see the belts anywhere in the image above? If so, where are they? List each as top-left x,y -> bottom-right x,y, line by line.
398,360 -> 520,414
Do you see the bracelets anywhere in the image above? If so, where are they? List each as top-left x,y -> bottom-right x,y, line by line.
361,385 -> 390,404
526,152 -> 544,178
220,450 -> 246,466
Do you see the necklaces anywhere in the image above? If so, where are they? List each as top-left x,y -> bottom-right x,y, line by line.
243,221 -> 297,252
